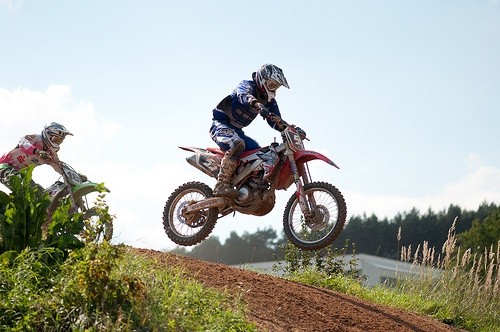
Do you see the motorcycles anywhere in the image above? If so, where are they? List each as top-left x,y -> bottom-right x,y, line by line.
34,153 -> 114,242
162,111 -> 347,250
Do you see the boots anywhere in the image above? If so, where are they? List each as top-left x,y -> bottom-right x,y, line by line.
213,150 -> 241,197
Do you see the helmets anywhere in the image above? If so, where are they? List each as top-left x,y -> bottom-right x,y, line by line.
41,122 -> 73,153
255,63 -> 290,102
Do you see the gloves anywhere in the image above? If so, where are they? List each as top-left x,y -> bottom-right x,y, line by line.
36,149 -> 49,160
295,127 -> 306,140
77,173 -> 87,182
258,104 -> 270,120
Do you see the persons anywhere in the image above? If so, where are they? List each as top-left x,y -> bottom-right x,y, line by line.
0,122 -> 87,199
209,63 -> 306,199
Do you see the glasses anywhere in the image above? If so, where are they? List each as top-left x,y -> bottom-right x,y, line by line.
268,80 -> 281,90
49,134 -> 64,146
